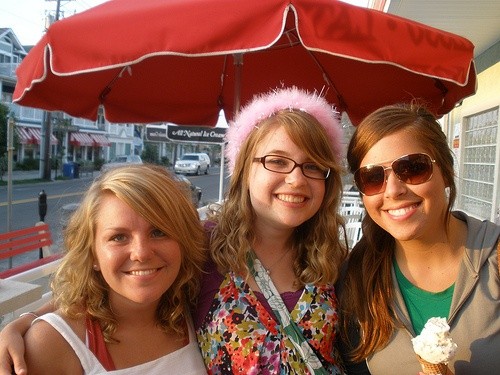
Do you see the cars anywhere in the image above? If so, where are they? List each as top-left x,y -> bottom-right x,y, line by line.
173,174 -> 202,208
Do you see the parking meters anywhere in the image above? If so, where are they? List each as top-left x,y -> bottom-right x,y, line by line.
39,190 -> 47,258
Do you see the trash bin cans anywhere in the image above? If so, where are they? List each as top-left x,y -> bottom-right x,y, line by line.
63,161 -> 74,178
73,161 -> 81,178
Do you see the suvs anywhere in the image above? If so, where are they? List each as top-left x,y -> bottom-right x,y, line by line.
175,152 -> 211,176
100,155 -> 143,174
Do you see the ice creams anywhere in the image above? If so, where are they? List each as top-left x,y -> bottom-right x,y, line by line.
411,316 -> 457,375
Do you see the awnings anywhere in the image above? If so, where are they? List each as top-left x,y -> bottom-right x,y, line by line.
16,127 -> 111,147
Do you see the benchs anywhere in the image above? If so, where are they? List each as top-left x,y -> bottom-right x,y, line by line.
0,224 -> 62,279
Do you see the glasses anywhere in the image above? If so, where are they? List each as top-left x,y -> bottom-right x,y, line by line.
252,155 -> 331,179
354,153 -> 441,196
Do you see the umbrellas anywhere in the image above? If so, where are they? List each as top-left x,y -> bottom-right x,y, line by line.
16,1 -> 479,126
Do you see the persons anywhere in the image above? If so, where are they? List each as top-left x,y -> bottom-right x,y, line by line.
335,103 -> 500,375
0,109 -> 349,375
22,166 -> 209,375
